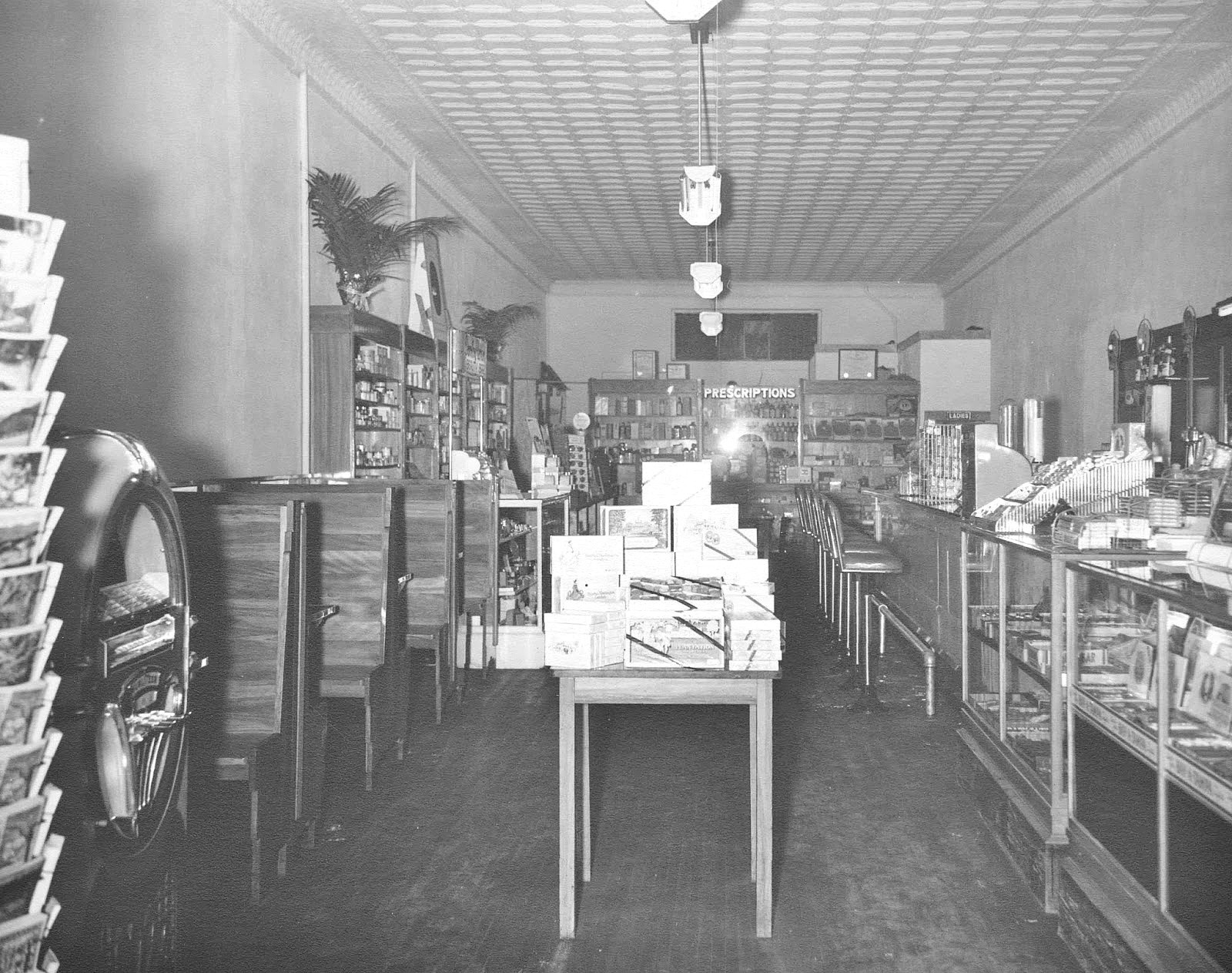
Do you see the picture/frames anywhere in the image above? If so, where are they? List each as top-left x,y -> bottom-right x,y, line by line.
838,349 -> 878,381
631,350 -> 657,379
666,362 -> 687,379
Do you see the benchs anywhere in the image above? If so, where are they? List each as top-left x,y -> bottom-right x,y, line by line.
338,477 -> 461,727
155,478 -> 310,908
258,482 -> 409,795
457,478 -> 501,682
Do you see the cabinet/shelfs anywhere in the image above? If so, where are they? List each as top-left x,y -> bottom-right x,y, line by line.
311,303 -> 516,486
797,378 -> 919,492
586,376 -> 704,496
956,522 -> 1232,973
707,380 -> 800,485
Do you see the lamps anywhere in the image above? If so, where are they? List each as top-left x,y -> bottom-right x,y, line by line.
678,22 -> 722,226
693,251 -> 724,300
690,225 -> 722,284
700,239 -> 725,338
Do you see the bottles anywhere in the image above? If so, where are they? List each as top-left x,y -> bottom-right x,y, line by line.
355,344 -> 505,469
594,396 -> 798,485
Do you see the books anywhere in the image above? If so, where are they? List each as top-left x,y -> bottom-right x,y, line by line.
545,504 -> 782,670
1054,497 -> 1184,549
0,212 -> 51,973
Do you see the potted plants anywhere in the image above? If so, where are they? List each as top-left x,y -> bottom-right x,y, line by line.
460,300 -> 543,366
306,166 -> 466,312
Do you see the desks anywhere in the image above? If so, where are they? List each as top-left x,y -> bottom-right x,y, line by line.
553,666 -> 782,938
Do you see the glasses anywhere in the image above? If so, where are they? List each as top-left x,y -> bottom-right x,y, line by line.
569,445 -> 588,490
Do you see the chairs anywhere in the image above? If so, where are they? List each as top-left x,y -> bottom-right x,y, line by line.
794,486 -> 906,687
